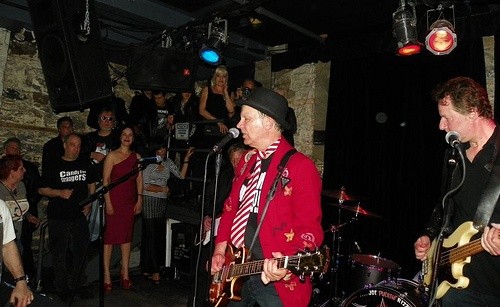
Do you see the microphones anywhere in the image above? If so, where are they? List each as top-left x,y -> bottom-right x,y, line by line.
136,155 -> 162,163
445,130 -> 461,148
212,128 -> 239,153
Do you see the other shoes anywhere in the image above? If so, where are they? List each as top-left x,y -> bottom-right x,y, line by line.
151,272 -> 161,281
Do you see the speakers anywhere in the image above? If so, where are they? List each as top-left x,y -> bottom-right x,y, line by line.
26,0 -> 114,114
125,42 -> 195,94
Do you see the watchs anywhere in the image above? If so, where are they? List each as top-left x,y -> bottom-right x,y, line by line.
15,274 -> 30,283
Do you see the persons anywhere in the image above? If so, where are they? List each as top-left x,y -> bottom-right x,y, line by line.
416,78 -> 500,307
0,67 -> 297,307
213,87 -> 323,306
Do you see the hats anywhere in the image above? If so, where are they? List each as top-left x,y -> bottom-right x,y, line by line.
238,87 -> 291,129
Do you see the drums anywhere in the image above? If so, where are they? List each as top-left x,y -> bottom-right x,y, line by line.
338,277 -> 429,307
336,253 -> 401,302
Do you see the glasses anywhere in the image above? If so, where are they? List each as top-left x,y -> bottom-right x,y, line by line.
99,116 -> 114,121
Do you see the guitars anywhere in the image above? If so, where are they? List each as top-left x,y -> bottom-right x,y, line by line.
421,220 -> 500,299
207,242 -> 331,307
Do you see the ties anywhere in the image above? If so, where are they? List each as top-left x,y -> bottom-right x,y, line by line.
230,136 -> 281,249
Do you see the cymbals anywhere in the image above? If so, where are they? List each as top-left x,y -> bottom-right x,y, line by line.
328,203 -> 386,220
322,189 -> 367,201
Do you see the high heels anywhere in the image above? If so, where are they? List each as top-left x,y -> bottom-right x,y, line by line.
104,277 -> 113,297
119,269 -> 132,290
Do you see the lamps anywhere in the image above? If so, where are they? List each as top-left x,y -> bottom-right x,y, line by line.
392,0 -> 423,57
199,17 -> 229,65
425,3 -> 458,55
